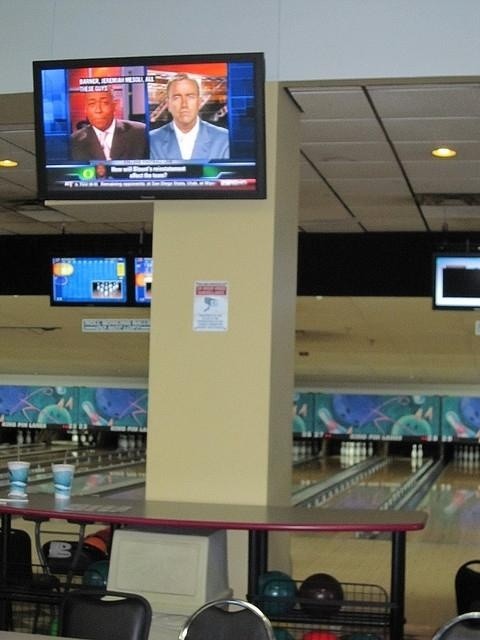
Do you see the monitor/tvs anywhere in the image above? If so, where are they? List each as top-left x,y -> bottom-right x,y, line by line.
130,255 -> 153,305
431,250 -> 479,311
31,51 -> 270,201
46,251 -> 129,307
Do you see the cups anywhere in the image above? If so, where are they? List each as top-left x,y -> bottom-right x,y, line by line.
7,461 -> 76,503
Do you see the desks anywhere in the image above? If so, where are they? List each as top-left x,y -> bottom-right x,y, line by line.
0,487 -> 428,639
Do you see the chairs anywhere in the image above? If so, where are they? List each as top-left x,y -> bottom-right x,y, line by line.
177,598 -> 276,639
0,528 -> 58,634
430,612 -> 480,640
56,589 -> 153,639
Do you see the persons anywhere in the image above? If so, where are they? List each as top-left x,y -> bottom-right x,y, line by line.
69,83 -> 147,160
149,76 -> 230,159
95,163 -> 115,180
